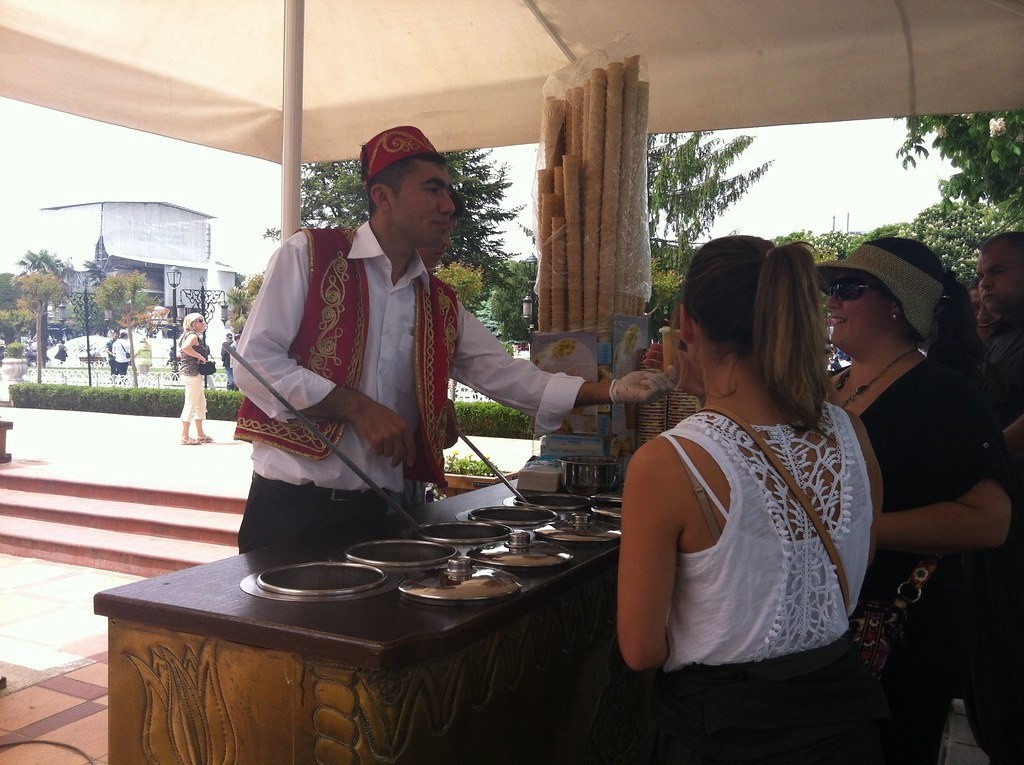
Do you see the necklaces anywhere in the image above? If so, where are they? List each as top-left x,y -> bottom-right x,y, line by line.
835,348 -> 916,409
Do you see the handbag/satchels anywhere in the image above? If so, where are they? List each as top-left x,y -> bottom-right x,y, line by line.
222,353 -> 229,366
849,595 -> 910,681
126,353 -> 130,358
198,362 -> 216,374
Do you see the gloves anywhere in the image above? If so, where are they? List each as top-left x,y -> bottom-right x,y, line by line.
609,368 -> 674,405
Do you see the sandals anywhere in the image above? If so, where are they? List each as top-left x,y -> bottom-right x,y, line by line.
181,437 -> 202,445
198,435 -> 213,443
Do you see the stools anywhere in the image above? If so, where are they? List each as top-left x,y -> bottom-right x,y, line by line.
0,421 -> 15,463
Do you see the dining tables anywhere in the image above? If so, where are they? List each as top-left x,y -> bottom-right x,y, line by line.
91,477 -> 658,765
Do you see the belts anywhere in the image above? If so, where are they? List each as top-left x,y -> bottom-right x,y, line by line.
254,471 -> 406,503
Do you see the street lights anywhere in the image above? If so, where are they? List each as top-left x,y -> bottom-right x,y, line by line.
58,303 -> 69,342
166,266 -> 184,381
523,254 -> 538,330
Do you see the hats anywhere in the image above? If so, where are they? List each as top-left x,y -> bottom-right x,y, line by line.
120,330 -> 127,336
360,126 -> 439,189
813,244 -> 945,341
226,333 -> 232,338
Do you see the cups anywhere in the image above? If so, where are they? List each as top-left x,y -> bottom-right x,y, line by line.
635,387 -> 702,451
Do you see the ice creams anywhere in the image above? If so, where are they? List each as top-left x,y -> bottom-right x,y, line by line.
658,327 -> 681,375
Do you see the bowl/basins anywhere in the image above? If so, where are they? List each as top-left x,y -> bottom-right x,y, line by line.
557,455 -> 619,498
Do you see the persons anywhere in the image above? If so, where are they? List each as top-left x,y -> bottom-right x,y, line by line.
165,347 -> 181,381
54,340 -> 68,365
105,329 -> 153,385
967,231 -> 1024,468
232,125 -> 678,555
177,312 -> 213,445
616,234 -> 884,765
221,332 -> 242,391
416,188 -> 465,449
642,237 -> 1023,765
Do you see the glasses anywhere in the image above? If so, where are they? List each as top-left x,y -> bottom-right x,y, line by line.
827,277 -> 883,300
195,319 -> 203,323
